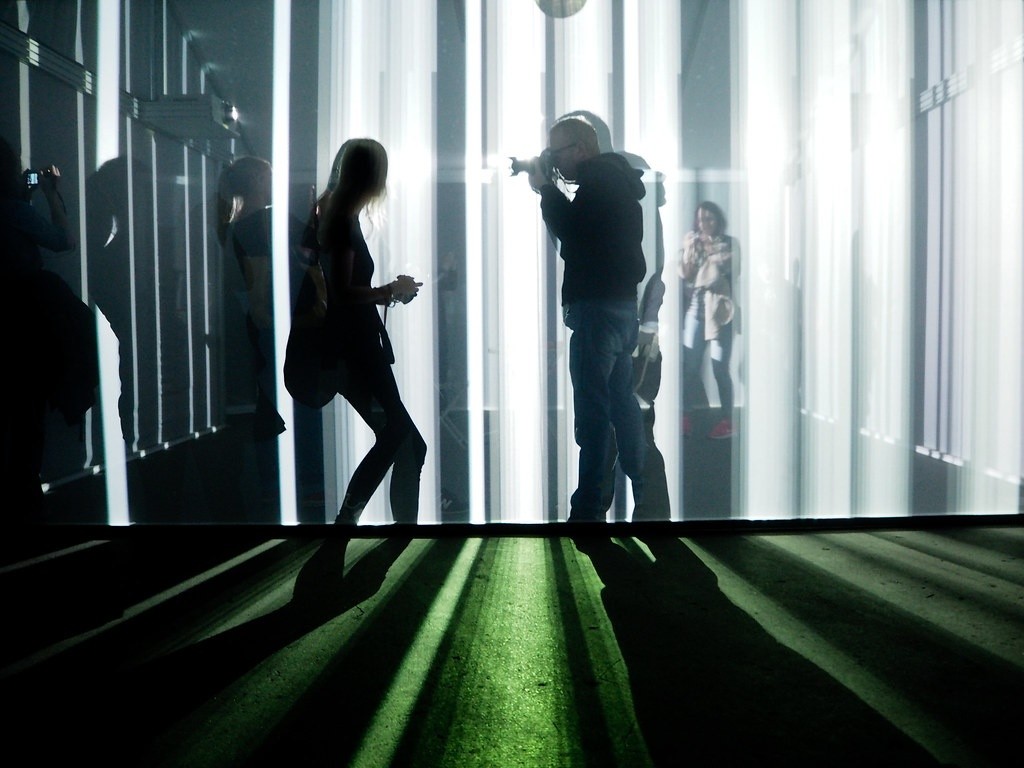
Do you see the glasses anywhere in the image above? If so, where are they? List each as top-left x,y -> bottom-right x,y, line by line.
547,143 -> 581,162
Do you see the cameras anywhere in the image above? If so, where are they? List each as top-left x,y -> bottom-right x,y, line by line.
509,147 -> 554,178
25,169 -> 52,188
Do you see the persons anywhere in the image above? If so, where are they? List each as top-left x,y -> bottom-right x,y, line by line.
306,139 -> 428,524
2,155 -> 271,524
677,201 -> 742,440
527,115 -> 671,523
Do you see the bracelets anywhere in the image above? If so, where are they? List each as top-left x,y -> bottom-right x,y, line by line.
381,284 -> 398,307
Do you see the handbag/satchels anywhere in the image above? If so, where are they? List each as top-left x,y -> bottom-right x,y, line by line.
283,238 -> 339,410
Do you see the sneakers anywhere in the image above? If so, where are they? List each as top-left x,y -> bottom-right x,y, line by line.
678,417 -> 693,436
709,419 -> 739,438
